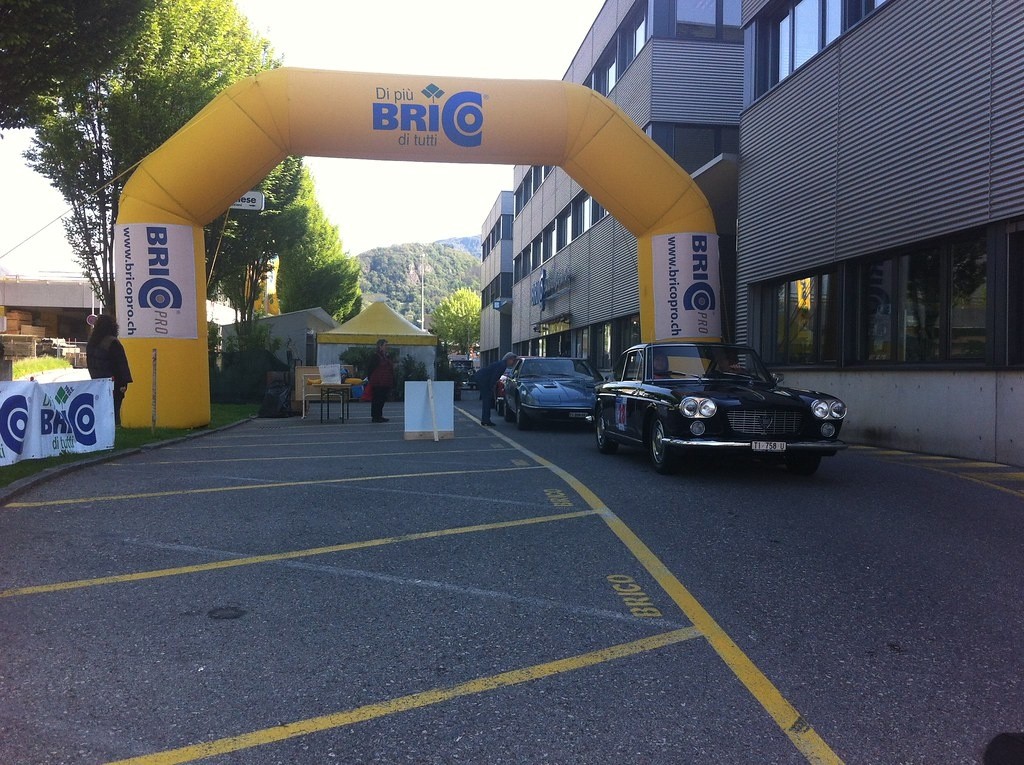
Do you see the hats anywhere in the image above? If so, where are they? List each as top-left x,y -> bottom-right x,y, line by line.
502,352 -> 517,361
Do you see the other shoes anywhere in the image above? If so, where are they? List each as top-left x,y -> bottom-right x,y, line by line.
372,417 -> 389,422
481,421 -> 496,426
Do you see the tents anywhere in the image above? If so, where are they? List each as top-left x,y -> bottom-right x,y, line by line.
316,300 -> 438,400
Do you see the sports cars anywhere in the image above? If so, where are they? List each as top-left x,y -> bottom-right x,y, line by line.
502,356 -> 606,431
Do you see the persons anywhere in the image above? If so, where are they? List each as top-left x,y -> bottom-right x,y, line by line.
648,351 -> 676,380
480,352 -> 517,426
85,315 -> 133,429
362,339 -> 394,423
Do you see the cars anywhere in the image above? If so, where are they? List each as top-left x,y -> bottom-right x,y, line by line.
590,340 -> 851,476
466,369 -> 480,390
479,367 -> 511,417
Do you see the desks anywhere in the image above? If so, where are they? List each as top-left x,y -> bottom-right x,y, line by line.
312,382 -> 352,424
